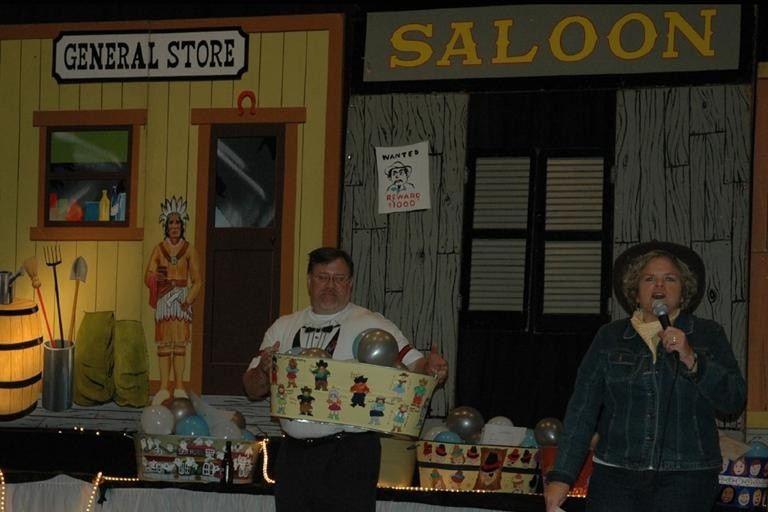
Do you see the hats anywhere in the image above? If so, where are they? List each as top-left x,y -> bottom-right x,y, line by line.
613,241 -> 706,316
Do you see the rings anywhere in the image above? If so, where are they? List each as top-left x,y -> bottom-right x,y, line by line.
672,337 -> 676,345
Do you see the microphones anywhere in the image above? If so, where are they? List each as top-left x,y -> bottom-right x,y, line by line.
651,299 -> 680,361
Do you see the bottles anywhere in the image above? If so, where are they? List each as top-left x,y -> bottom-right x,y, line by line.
221,439 -> 234,485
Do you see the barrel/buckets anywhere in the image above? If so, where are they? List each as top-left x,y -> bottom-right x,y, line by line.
0,295 -> 44,422
43,339 -> 76,413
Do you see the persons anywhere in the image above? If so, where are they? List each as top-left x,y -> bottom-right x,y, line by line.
242,248 -> 448,512
144,196 -> 202,406
384,162 -> 414,192
544,242 -> 748,512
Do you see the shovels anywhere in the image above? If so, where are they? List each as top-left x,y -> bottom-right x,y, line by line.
66,255 -> 88,347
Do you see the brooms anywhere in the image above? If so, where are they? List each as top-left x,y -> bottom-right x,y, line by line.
21,254 -> 56,348
